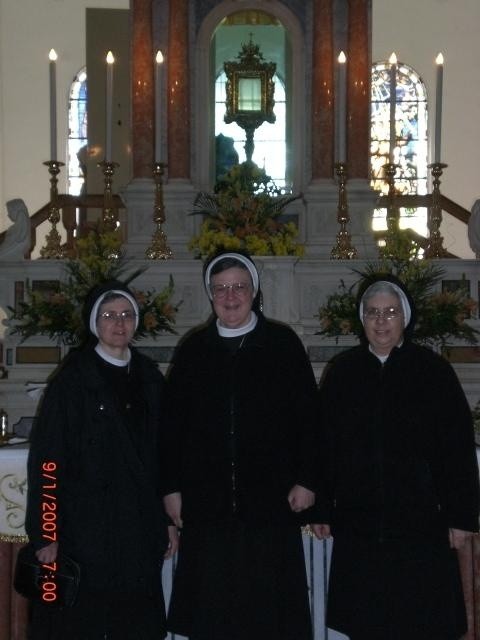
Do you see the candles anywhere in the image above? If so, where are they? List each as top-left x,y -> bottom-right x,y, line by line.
334,49 -> 348,162
389,48 -> 398,164
433,51 -> 444,162
154,49 -> 165,163
106,50 -> 114,162
48,46 -> 58,157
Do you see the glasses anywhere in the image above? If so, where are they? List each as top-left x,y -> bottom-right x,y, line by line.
364,307 -> 403,321
98,311 -> 134,321
210,284 -> 251,297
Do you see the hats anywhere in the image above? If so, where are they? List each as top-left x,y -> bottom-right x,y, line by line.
204,252 -> 259,300
82,280 -> 140,337
356,274 -> 414,330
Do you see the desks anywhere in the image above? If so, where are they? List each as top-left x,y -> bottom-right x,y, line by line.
0,442 -> 479,639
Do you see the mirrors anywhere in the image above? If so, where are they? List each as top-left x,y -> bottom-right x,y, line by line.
211,22 -> 287,197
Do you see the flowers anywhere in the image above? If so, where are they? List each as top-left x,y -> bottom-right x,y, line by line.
184,177 -> 307,261
1,230 -> 187,346
217,156 -> 276,195
308,246 -> 478,348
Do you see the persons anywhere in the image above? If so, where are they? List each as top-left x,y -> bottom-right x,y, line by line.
308,272 -> 480,640
149,247 -> 320,639
24,277 -> 181,640
0,198 -> 32,260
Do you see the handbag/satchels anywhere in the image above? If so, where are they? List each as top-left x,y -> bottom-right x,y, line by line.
13,544 -> 80,609
